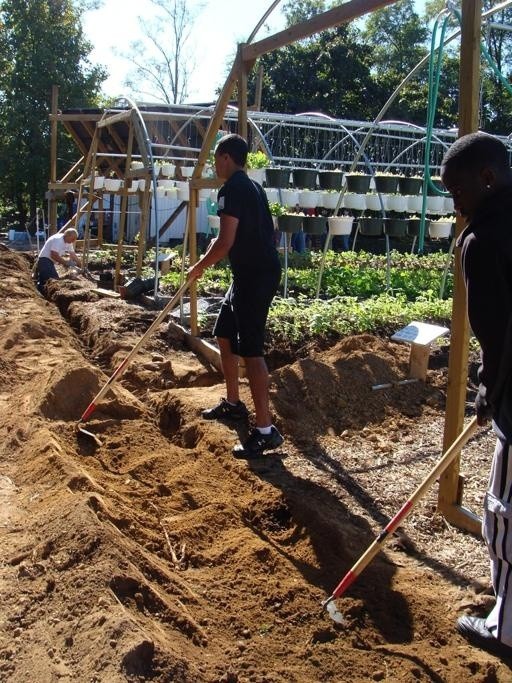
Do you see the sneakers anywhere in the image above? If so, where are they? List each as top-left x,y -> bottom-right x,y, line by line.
201,399 -> 248,419
232,425 -> 283,457
457,616 -> 512,654
37,281 -> 43,287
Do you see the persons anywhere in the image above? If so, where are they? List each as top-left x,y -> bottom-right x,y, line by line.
186,133 -> 285,459
38,228 -> 81,286
440,132 -> 512,666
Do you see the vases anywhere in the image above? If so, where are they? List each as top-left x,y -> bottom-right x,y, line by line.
88,176 -> 211,200
131,162 -> 195,177
206,215 -> 220,228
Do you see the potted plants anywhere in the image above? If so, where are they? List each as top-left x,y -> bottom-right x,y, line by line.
245,151 -> 456,238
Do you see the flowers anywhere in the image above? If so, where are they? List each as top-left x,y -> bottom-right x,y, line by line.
91,165 -> 99,175
109,169 -> 115,178
